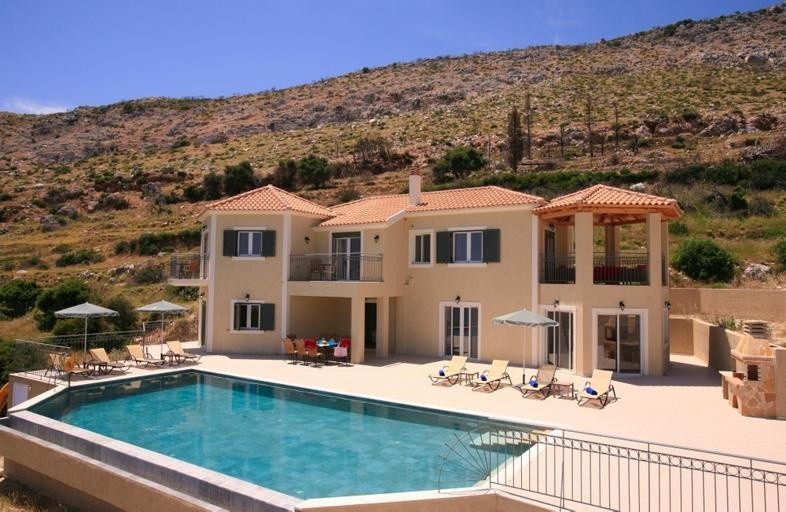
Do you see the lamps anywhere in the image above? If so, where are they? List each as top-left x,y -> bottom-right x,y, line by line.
455,296 -> 460,304
374,235 -> 379,242
668,302 -> 671,310
304,236 -> 310,244
620,301 -> 624,312
246,294 -> 250,301
552,299 -> 559,307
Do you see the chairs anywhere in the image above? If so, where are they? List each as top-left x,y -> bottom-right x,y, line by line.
165,340 -> 197,364
575,369 -> 617,408
282,333 -> 351,366
88,348 -> 132,374
44,352 -> 90,381
471,359 -> 512,391
126,344 -> 165,368
310,258 -> 324,279
520,363 -> 558,400
323,259 -> 337,279
428,356 -> 468,385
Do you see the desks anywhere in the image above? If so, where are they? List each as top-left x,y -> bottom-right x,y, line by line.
551,381 -> 574,398
459,371 -> 479,387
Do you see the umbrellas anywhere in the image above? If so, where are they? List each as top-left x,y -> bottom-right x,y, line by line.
490,308 -> 560,383
133,298 -> 190,354
53,302 -> 119,358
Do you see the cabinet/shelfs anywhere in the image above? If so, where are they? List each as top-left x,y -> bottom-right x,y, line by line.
596,338 -> 640,373
